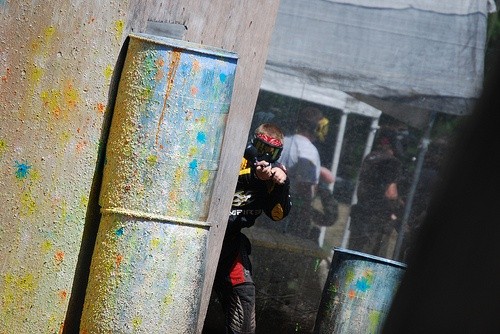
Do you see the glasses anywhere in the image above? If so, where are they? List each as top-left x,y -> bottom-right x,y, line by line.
251,134 -> 282,160
314,118 -> 330,141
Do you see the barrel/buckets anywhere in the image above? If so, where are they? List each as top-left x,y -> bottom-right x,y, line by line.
99,33 -> 239,224
312,246 -> 408,334
79,208 -> 209,334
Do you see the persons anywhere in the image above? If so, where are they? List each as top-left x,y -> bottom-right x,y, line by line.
215,123 -> 290,334
248,100 -> 444,263
